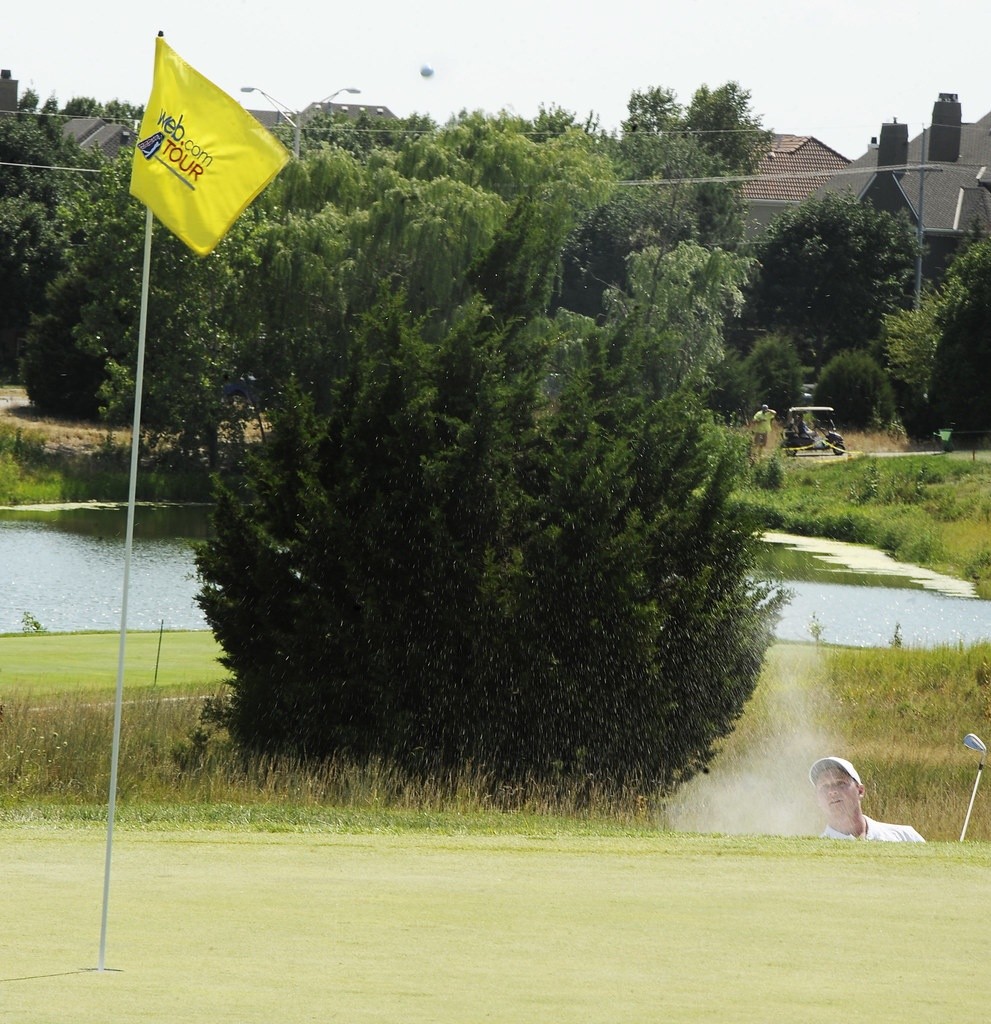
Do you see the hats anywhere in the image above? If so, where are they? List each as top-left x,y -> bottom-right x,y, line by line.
809,756 -> 863,786
762,404 -> 768,408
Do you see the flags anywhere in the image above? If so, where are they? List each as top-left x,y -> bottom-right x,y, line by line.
128,39 -> 289,258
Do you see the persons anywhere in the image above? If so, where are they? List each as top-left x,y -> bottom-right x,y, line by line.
798,414 -> 818,438
810,756 -> 926,843
753,404 -> 777,457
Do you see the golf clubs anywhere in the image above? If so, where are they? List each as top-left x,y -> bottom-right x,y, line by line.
959,732 -> 987,842
